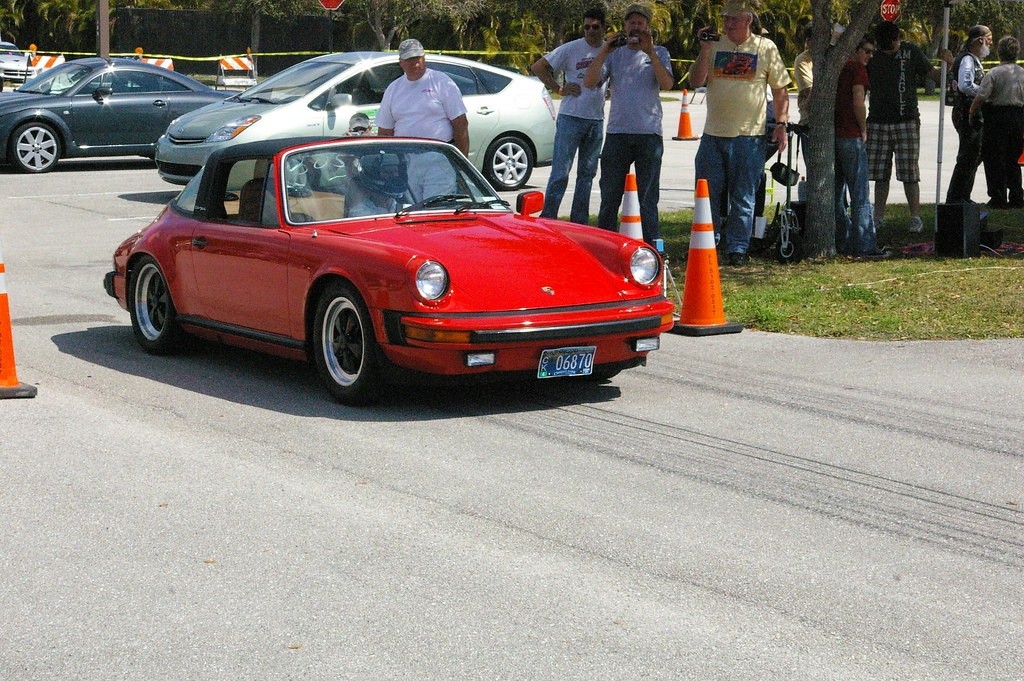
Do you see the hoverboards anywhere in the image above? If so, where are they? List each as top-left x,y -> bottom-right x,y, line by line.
747,121 -> 812,265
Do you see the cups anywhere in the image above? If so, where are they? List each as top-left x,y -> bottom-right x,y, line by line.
754,216 -> 767,239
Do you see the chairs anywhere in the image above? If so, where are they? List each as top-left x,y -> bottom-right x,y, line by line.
238,176 -> 278,227
140,74 -> 163,92
342,176 -> 398,218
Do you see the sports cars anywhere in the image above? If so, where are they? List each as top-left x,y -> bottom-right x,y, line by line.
101,135 -> 676,406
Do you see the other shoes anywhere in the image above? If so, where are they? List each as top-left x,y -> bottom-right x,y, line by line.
874,217 -> 886,230
864,249 -> 893,258
966,198 -> 975,204
946,199 -> 969,204
909,215 -> 924,233
727,252 -> 745,266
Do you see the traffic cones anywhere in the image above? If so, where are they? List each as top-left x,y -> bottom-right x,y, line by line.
662,179 -> 745,337
618,173 -> 644,243
672,88 -> 700,141
0,261 -> 38,398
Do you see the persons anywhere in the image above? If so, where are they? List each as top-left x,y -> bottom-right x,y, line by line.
795,17 -> 1024,256
688,0 -> 792,266
583,5 -> 675,255
344,112 -> 374,178
377,39 -> 469,210
531,8 -> 613,223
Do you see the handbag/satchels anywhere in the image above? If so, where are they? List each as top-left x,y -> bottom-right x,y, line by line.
770,162 -> 800,186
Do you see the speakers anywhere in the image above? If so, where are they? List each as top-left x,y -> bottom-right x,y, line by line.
936,203 -> 980,258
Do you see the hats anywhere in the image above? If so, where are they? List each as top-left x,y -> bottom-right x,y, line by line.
719,0 -> 751,18
349,113 -> 370,128
625,3 -> 652,23
399,39 -> 425,59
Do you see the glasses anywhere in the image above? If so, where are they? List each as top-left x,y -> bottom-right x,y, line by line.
584,24 -> 600,30
861,47 -> 875,55
354,127 -> 367,132
973,36 -> 992,43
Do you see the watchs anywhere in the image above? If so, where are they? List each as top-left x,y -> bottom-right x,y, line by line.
776,122 -> 788,128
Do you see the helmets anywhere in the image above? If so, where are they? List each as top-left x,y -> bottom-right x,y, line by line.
351,149 -> 410,199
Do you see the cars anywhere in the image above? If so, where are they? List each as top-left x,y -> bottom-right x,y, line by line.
0,56 -> 245,174
153,51 -> 559,194
0,41 -> 37,83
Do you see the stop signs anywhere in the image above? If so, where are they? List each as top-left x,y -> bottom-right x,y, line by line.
881,0 -> 903,23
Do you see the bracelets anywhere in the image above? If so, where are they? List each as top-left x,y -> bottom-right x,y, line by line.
558,86 -> 562,94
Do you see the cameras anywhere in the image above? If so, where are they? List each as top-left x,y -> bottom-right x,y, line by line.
349,132 -> 360,136
608,28 -> 640,48
700,27 -> 720,41
